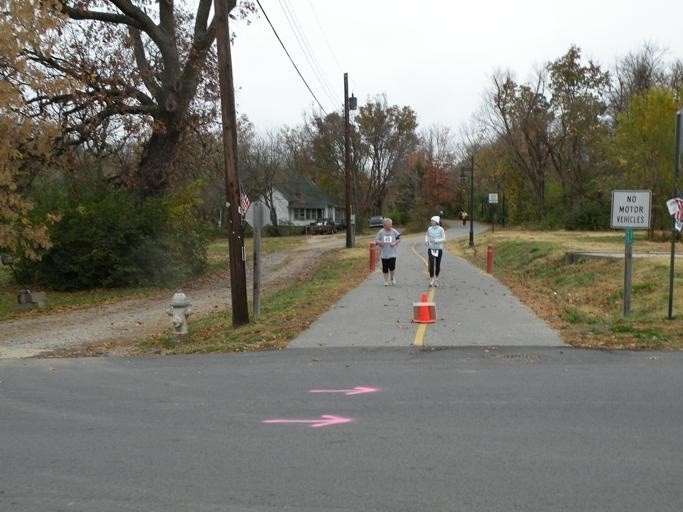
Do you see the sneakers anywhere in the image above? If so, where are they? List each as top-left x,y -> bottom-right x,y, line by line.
429,279 -> 438,287
384,280 -> 396,286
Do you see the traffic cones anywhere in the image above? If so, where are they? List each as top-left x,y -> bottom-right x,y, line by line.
414,293 -> 436,322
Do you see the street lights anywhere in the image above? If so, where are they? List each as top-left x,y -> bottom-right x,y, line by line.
458,153 -> 475,248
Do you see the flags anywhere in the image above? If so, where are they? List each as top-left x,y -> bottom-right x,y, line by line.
240,183 -> 250,215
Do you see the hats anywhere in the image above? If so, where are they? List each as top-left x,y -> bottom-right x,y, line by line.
431,216 -> 440,225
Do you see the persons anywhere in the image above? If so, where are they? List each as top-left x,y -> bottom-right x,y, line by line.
425,215 -> 446,287
462,210 -> 468,226
375,218 -> 401,286
